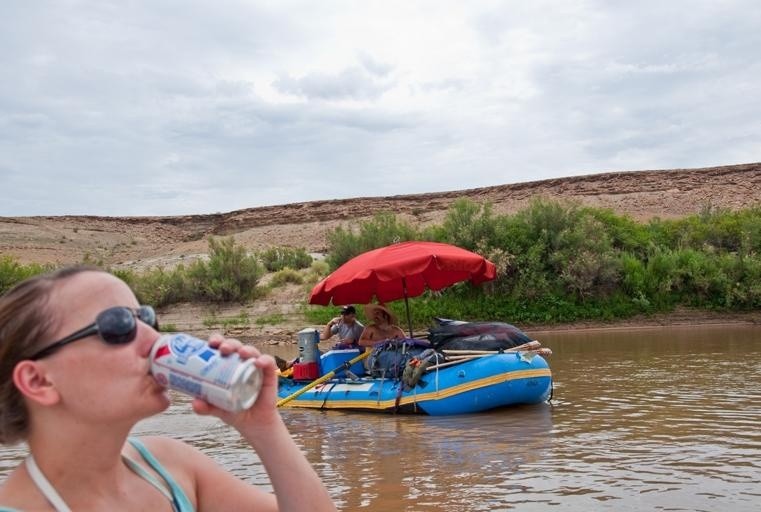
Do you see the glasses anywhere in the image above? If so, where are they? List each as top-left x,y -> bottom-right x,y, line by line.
29,305 -> 157,360
341,312 -> 349,315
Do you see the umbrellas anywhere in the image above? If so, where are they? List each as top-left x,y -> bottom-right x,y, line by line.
308,241 -> 496,340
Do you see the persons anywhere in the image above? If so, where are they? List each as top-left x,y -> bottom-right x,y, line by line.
0,266 -> 337,512
323,306 -> 406,354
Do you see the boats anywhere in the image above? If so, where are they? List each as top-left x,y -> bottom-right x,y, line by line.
276,349 -> 553,416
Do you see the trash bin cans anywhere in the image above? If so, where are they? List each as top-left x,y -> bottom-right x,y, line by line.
298,327 -> 320,363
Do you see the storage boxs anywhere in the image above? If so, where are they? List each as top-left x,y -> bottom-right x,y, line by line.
319,349 -> 366,377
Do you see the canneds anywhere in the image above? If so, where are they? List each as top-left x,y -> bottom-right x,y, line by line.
336,319 -> 344,328
150,333 -> 262,412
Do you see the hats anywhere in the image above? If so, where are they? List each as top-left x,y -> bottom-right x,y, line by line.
366,303 -> 398,323
338,305 -> 355,313
